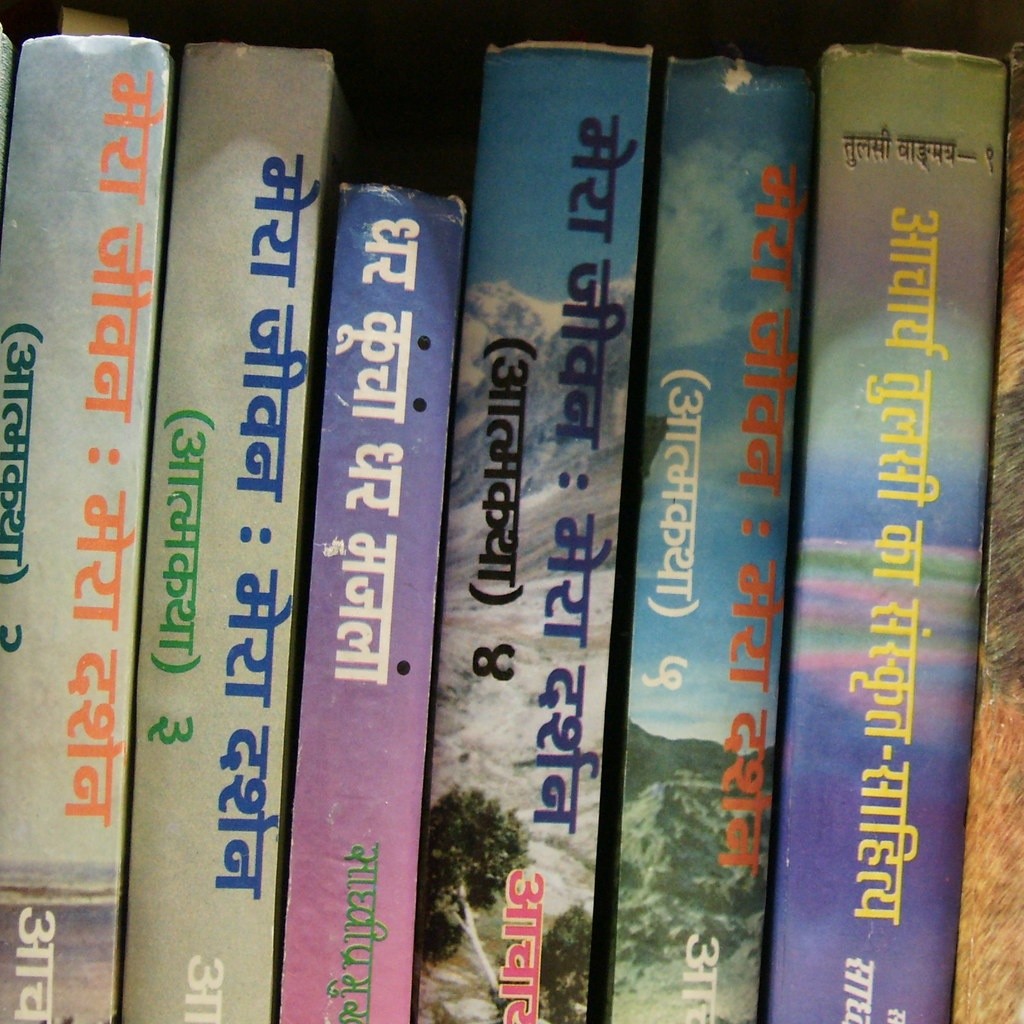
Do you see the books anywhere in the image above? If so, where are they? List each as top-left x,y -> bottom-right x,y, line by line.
281,176 -> 465,1023
418,39 -> 654,1023
609,60 -> 813,1021
764,39 -> 1004,1024
0,32 -> 173,1024
121,44 -> 335,1023
